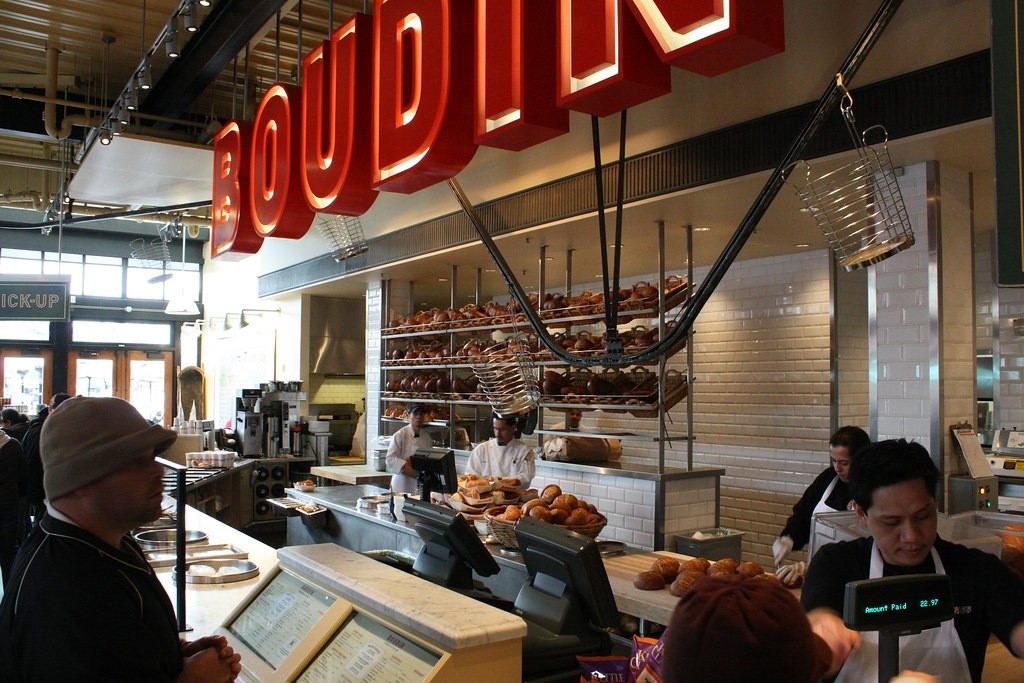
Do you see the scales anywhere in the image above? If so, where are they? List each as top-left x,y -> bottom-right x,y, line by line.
844,574 -> 954,683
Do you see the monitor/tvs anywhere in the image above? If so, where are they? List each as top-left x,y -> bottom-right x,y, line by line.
514,516 -> 622,629
400,498 -> 502,577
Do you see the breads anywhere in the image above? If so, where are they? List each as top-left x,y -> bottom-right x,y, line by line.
632,557 -> 780,598
381,284 -> 678,423
448,474 -> 604,527
191,460 -> 209,467
1001,524 -> 1024,574
302,479 -> 314,486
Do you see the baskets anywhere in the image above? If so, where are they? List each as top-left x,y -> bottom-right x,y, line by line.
484,504 -> 608,549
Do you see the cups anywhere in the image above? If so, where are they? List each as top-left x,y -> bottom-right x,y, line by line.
173,417 -> 203,434
186,450 -> 235,468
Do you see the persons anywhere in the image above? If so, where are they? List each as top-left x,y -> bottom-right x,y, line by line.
465,416 -> 535,488
661,574 -> 833,683
800,439 -> 1024,682
0,393 -> 71,593
385,405 -> 432,494
0,394 -> 243,683
772,425 -> 873,585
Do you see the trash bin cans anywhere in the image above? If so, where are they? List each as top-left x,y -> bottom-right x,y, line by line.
672,528 -> 746,565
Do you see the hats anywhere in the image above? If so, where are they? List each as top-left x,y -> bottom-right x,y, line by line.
411,403 -> 426,413
40,394 -> 177,501
660,573 -> 834,683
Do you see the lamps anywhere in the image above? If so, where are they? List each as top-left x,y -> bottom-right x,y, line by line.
180,309 -> 281,381
165,226 -> 200,315
41,191 -> 71,235
98,0 -> 213,145
164,215 -> 183,243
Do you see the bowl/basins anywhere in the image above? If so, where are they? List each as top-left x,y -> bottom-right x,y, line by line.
308,420 -> 329,433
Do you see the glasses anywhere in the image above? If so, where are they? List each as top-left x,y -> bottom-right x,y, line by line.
0,419 -> 9,424
